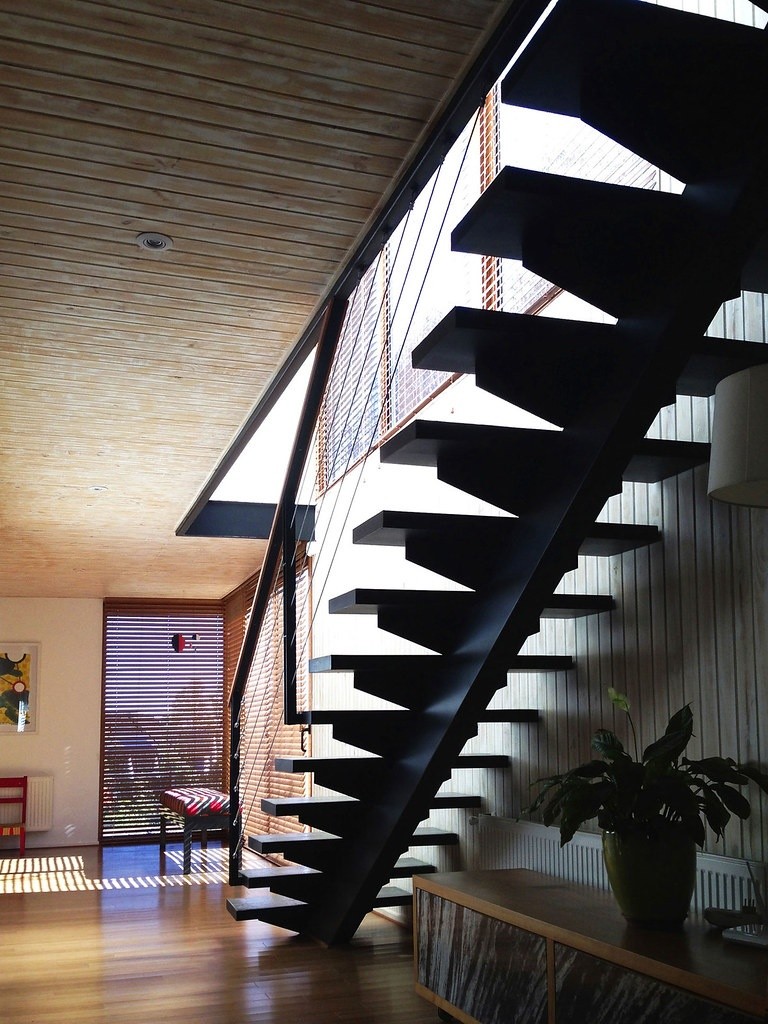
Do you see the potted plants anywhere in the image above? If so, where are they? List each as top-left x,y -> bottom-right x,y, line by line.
514,686 -> 768,934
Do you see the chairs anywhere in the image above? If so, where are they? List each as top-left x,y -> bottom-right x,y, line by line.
0,776 -> 28,856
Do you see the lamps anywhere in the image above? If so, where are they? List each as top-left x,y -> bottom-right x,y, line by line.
707,363 -> 768,509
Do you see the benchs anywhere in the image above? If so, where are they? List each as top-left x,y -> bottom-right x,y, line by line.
156,787 -> 243,876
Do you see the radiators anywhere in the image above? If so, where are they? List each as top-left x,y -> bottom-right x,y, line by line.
477,813 -> 766,943
0,776 -> 54,831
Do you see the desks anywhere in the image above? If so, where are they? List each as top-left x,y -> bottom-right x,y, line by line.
411,868 -> 768,1024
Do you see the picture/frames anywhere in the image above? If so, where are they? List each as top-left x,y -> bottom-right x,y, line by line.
0,642 -> 43,735
